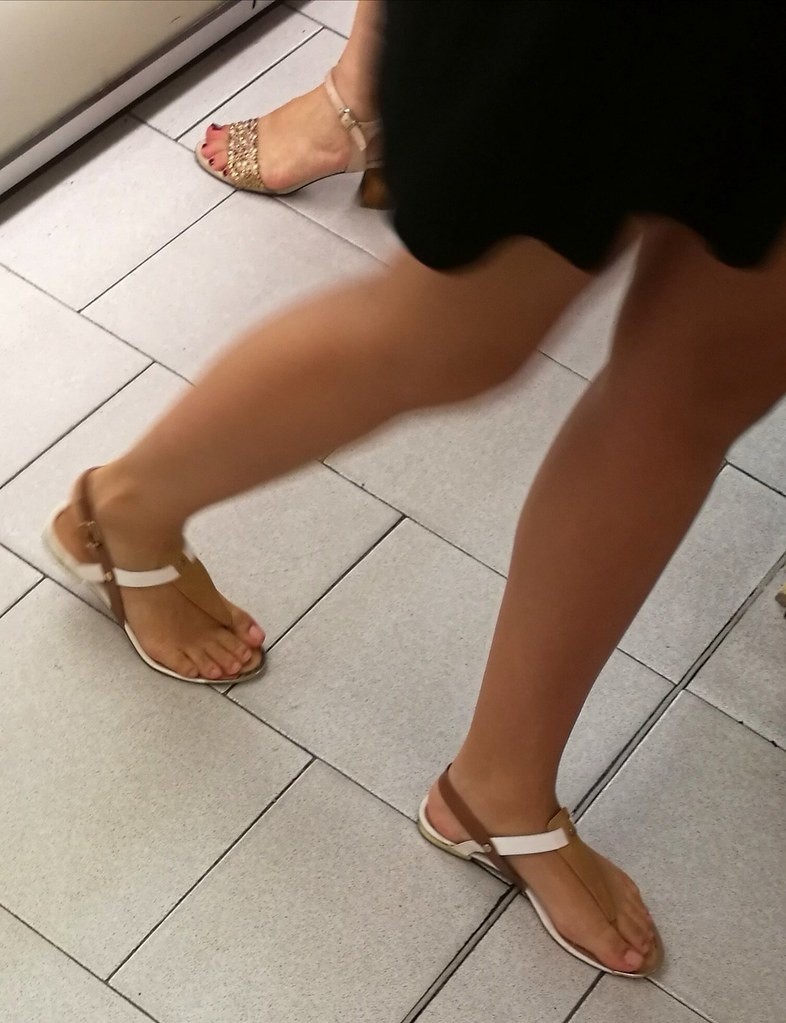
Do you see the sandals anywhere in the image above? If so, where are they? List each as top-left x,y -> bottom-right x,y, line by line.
41,465 -> 267,684
417,762 -> 665,977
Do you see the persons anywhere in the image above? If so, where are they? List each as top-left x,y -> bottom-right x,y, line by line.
44,0 -> 786,978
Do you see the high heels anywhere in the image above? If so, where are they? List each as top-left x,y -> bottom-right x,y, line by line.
194,66 -> 392,210
